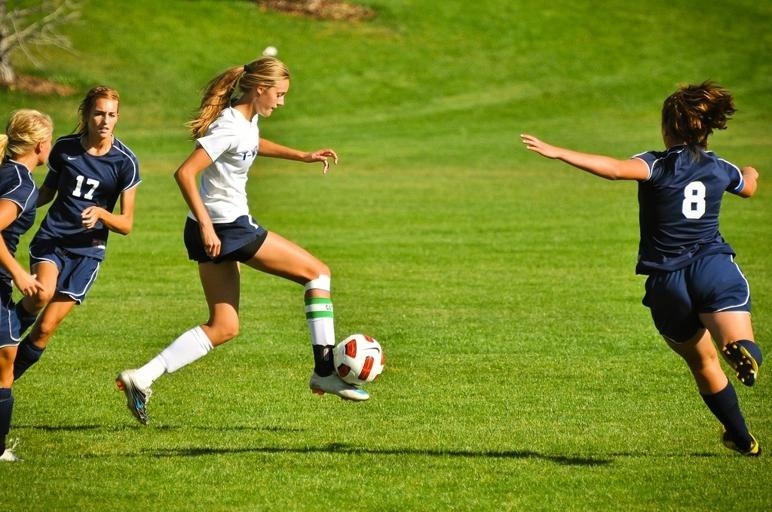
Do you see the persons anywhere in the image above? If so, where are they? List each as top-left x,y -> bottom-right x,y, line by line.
521,78 -> 763,456
17,85 -> 142,379
117,55 -> 385,423
2,109 -> 53,461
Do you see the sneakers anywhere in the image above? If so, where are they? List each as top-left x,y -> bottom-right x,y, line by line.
310,371 -> 370,401
116,369 -> 152,426
720,339 -> 762,387
1,448 -> 27,464
718,424 -> 761,456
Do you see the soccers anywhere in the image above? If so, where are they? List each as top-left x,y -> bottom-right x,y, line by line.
334,334 -> 385,386
